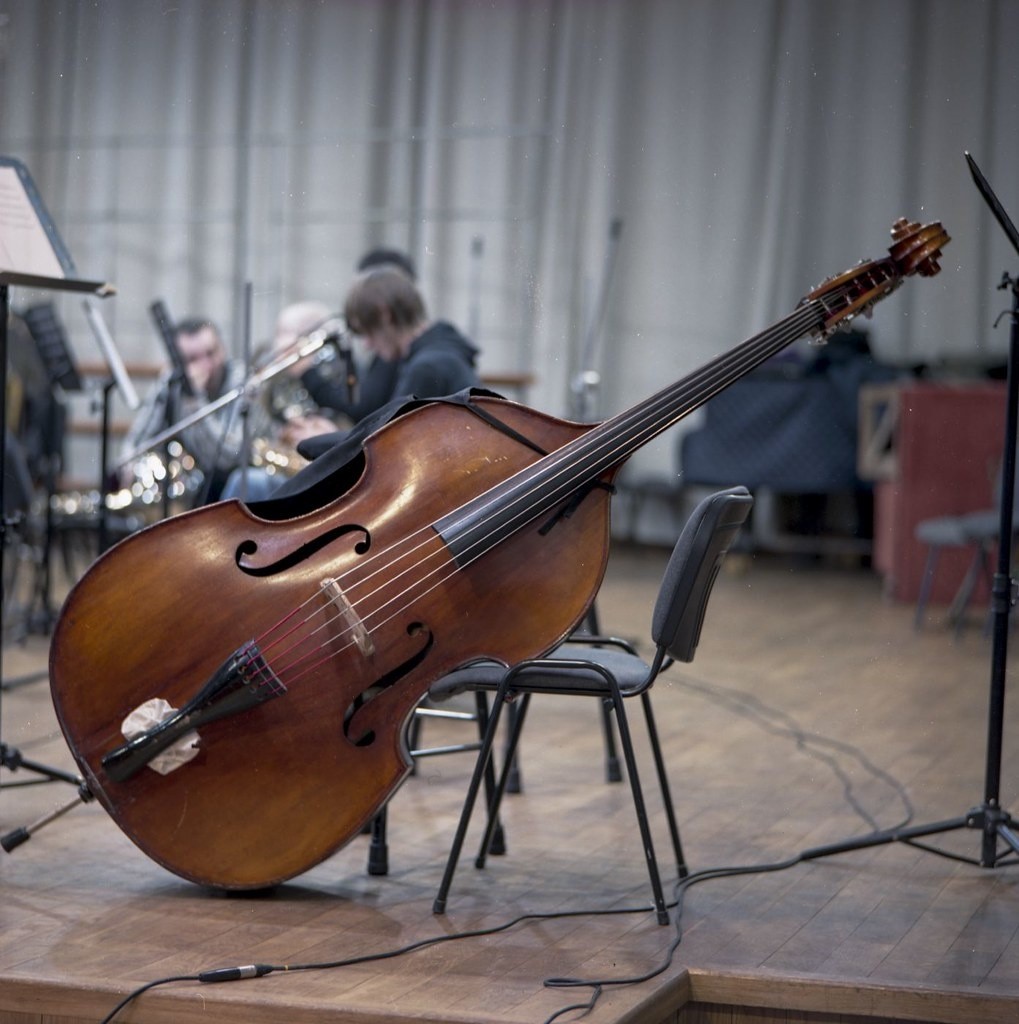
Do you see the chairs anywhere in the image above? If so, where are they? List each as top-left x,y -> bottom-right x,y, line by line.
916,439 -> 1000,626
356,483 -> 755,929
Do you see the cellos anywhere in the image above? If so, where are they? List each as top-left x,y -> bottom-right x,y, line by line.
0,212 -> 954,893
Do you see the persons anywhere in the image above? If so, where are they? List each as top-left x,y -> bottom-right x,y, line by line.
124,249 -> 482,513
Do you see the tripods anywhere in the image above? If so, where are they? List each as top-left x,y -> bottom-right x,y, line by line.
800,149 -> 1019,869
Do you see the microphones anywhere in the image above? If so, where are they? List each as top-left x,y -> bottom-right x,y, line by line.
340,349 -> 356,386
